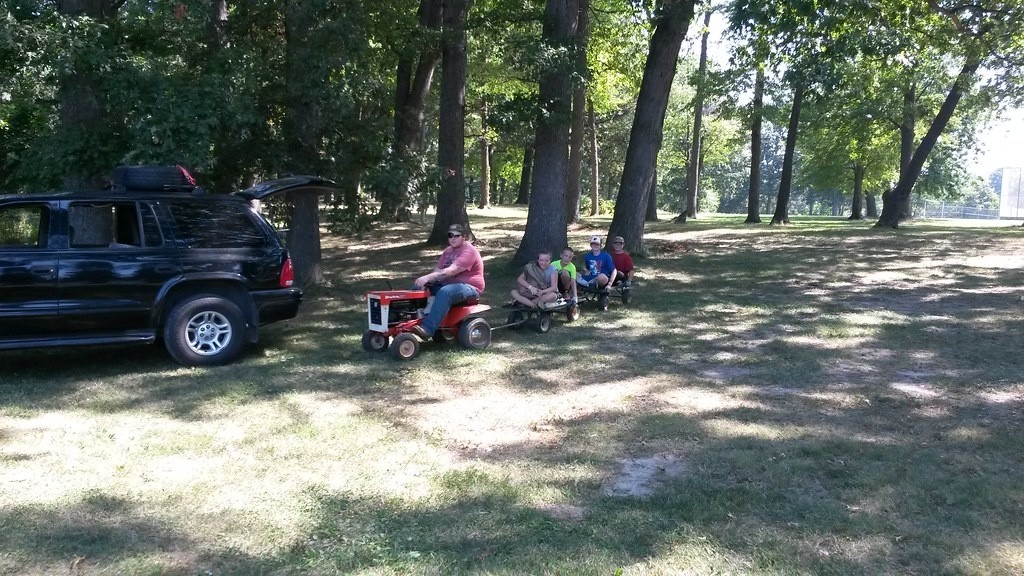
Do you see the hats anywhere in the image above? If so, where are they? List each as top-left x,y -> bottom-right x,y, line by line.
613,236 -> 624,244
590,236 -> 602,247
449,224 -> 465,233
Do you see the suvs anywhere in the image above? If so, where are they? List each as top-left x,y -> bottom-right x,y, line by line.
0,165 -> 345,367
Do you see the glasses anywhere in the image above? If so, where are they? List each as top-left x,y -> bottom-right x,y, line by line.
448,233 -> 462,238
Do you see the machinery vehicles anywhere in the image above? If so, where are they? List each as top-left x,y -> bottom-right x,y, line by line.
362,282 -> 636,361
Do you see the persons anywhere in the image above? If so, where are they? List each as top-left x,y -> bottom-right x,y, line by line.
550,247 -> 578,303
511,249 -> 558,309
577,236 -> 617,292
411,224 -> 485,341
608,234 -> 634,287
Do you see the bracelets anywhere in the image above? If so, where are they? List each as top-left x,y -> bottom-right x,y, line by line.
609,282 -> 612,285
528,285 -> 532,289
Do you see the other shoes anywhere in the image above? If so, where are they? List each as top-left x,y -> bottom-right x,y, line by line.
563,289 -> 571,301
412,325 -> 429,341
587,282 -> 599,289
577,284 -> 586,292
536,302 -> 545,310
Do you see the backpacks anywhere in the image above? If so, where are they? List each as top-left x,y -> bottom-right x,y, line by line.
520,261 -> 547,299
